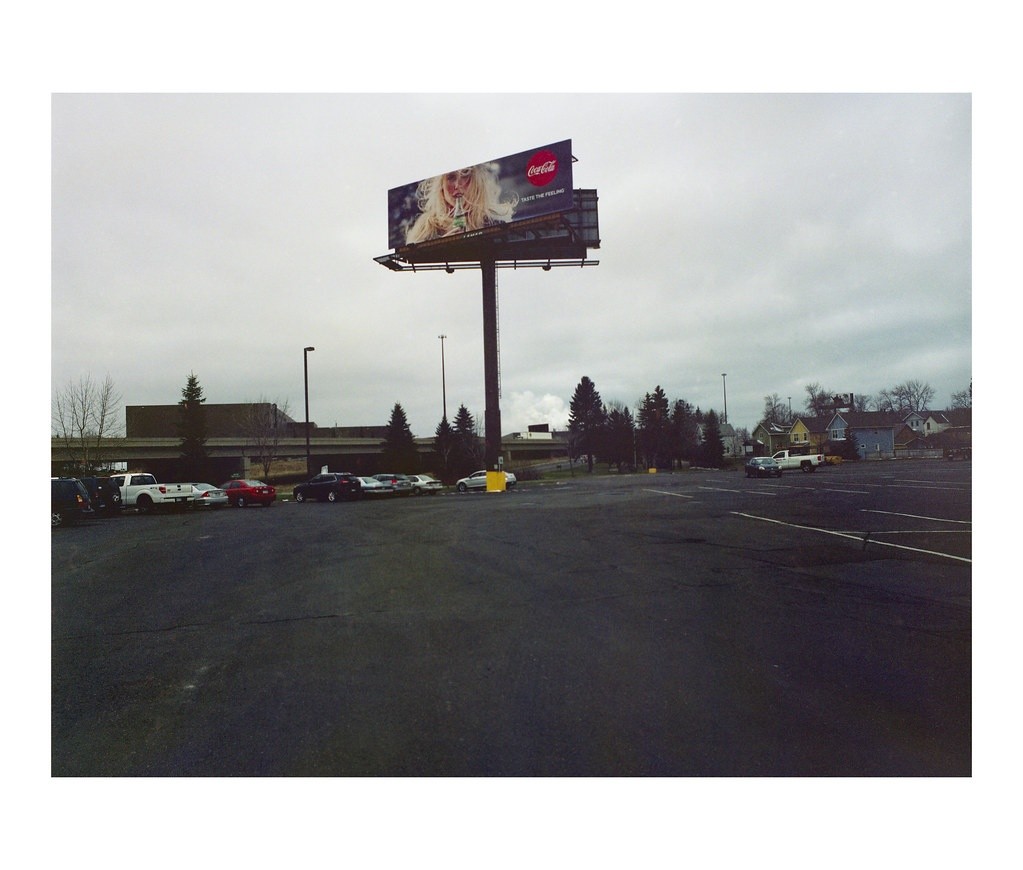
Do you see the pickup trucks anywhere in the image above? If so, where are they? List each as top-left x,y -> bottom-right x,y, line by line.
772,450 -> 825,473
107,473 -> 195,516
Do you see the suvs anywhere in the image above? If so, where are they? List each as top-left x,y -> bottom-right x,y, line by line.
78,476 -> 122,518
292,472 -> 360,503
51,477 -> 95,526
373,473 -> 413,498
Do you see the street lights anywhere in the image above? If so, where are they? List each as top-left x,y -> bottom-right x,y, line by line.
436,333 -> 448,423
304,345 -> 315,483
719,372 -> 728,423
788,396 -> 791,418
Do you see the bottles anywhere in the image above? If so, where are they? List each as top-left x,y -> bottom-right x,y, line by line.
454,196 -> 466,234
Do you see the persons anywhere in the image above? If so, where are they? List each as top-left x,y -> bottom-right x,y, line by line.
406,164 -> 512,245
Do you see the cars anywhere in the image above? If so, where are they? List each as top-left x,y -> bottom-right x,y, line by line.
454,470 -> 517,492
357,476 -> 394,498
190,484 -> 230,512
219,480 -> 277,508
405,474 -> 443,496
746,456 -> 783,479
